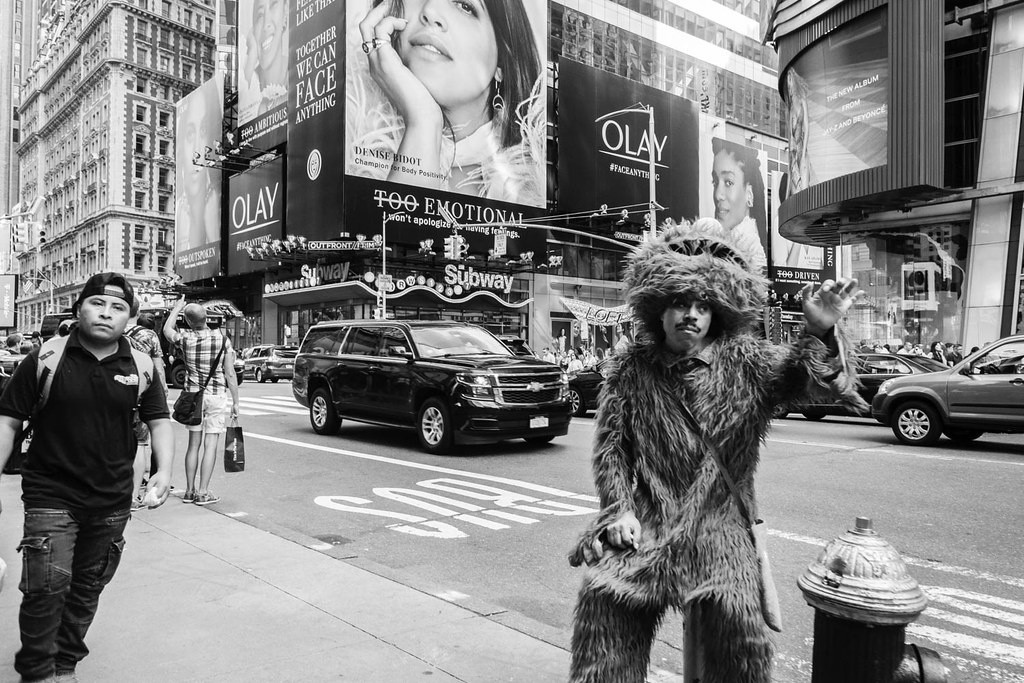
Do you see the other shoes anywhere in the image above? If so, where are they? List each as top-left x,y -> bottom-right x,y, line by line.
170,486 -> 174,490
34,673 -> 78,683
140,477 -> 149,488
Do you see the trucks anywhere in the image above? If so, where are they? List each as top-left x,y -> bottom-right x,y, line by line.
140,306 -> 245,389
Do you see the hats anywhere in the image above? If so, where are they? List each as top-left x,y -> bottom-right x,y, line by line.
78,272 -> 133,307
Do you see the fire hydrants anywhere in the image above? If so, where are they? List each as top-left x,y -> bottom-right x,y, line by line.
792,514 -> 952,683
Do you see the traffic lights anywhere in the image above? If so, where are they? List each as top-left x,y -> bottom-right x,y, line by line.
16,222 -> 30,246
11,225 -> 25,255
39,229 -> 49,245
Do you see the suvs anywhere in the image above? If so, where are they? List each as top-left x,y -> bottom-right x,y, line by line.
292,317 -> 574,456
243,344 -> 299,383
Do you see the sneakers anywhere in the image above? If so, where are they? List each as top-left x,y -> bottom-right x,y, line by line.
194,490 -> 220,505
182,490 -> 196,502
130,494 -> 146,511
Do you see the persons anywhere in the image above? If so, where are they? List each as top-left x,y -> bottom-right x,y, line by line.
0,272 -> 175,683
533,322 -> 636,373
164,293 -> 240,507
175,75 -> 226,253
560,219 -> 865,683
239,0 -> 289,124
848,338 -> 992,375
711,136 -> 768,267
359,0 -> 547,209
783,68 -> 819,201
0,311 -> 174,514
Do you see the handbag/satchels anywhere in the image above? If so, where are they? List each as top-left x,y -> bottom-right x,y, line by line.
223,415 -> 245,472
747,526 -> 782,633
172,389 -> 203,426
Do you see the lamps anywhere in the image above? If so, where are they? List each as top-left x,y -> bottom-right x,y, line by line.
549,256 -> 563,267
373,235 -> 382,248
356,234 -> 366,247
419,239 -> 434,253
520,252 -> 534,263
193,133 -> 279,173
245,235 -> 307,259
488,249 -> 500,260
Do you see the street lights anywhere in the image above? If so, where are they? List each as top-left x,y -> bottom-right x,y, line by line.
381,210 -> 406,320
594,101 -> 656,239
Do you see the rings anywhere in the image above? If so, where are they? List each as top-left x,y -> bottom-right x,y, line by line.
372,37 -> 390,50
362,41 -> 373,55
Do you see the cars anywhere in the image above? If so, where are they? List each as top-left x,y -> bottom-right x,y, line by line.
870,333 -> 1024,447
799,353 -> 953,421
567,353 -> 613,417
